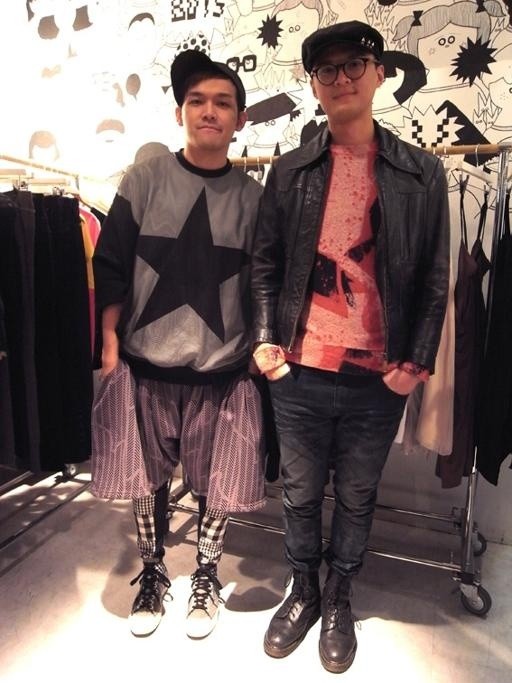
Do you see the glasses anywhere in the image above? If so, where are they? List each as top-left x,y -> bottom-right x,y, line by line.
312,58 -> 379,86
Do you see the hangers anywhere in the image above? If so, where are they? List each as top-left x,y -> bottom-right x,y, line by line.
230,144 -> 512,193
0,153 -> 92,209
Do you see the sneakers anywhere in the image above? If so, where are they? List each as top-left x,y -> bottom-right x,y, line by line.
185,570 -> 219,638
128,570 -> 171,636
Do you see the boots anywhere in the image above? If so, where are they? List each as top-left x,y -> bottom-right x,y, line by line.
264,568 -> 358,673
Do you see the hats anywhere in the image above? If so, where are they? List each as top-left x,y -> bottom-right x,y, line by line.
170,49 -> 246,112
302,20 -> 384,80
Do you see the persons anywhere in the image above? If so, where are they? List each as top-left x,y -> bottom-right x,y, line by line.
91,47 -> 267,640
252,18 -> 452,676
